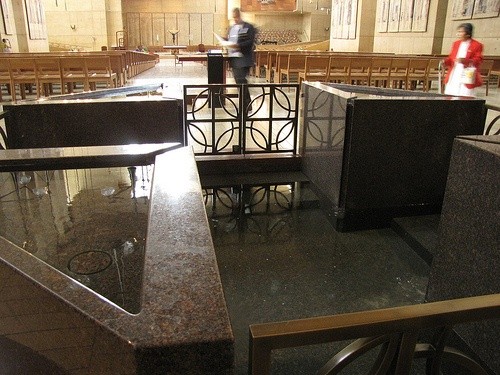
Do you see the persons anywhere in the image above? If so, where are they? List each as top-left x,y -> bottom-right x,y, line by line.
440,23 -> 483,97
220,8 -> 255,114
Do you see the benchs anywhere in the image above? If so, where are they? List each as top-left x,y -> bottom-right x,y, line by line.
249,49 -> 500,95
0,49 -> 159,99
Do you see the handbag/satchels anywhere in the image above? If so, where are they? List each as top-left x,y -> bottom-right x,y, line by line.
462,59 -> 476,84
